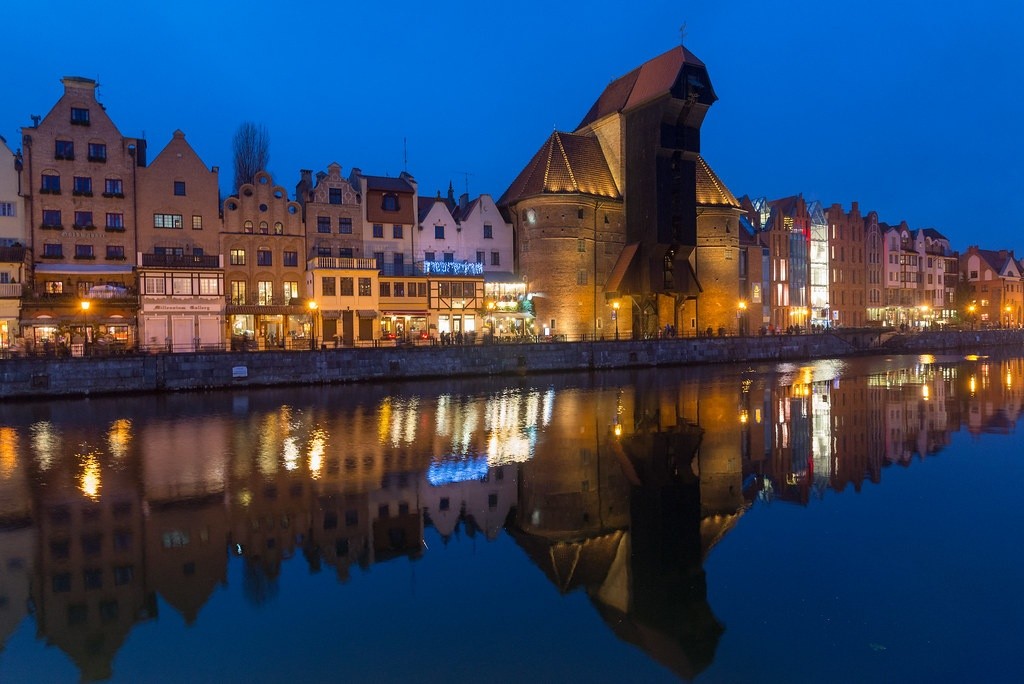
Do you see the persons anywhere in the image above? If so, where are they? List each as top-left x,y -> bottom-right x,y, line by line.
706,323 -> 729,338
663,323 -> 677,339
809,322 -> 825,334
437,330 -> 469,347
756,324 -> 780,337
786,323 -> 804,335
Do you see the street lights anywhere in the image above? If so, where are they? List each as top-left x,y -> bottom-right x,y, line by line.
970,306 -> 974,330
613,302 -> 619,340
489,302 -> 493,335
1007,306 -> 1010,329
81,301 -> 90,353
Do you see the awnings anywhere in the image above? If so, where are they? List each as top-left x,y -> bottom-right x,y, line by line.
357,310 -> 377,319
323,312 -> 340,320
